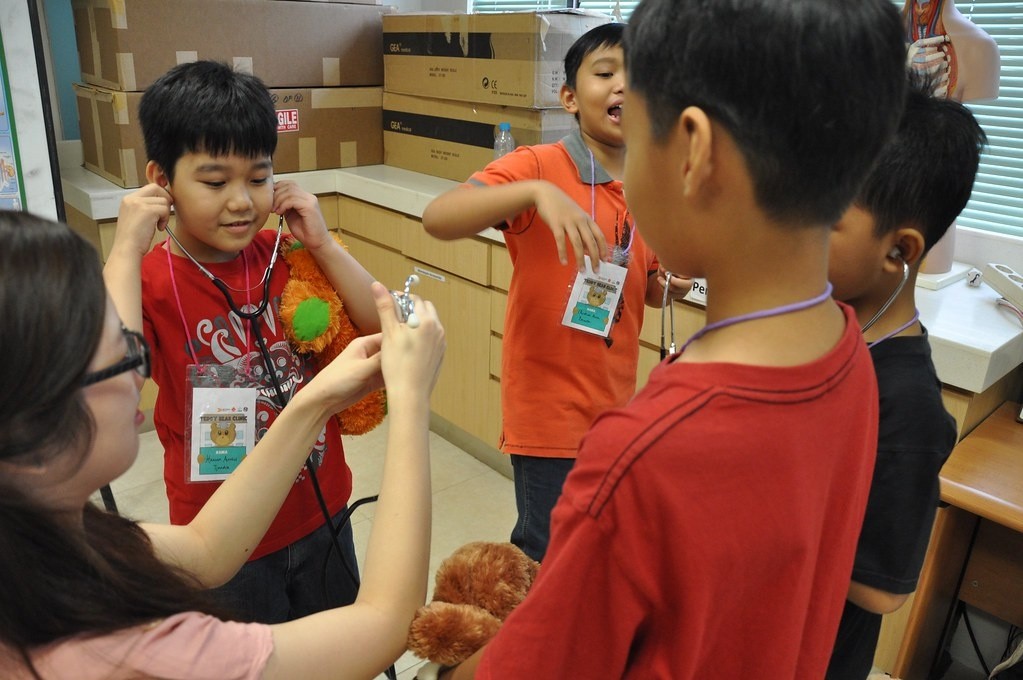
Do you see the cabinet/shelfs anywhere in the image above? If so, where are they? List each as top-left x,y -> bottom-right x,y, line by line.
56,139 -> 1013,680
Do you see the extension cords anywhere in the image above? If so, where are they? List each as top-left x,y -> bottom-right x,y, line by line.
984,264 -> 1023,312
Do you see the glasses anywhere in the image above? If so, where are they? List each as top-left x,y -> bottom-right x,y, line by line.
79,324 -> 151,387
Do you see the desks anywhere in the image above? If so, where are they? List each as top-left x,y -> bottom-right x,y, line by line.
887,390 -> 1023,680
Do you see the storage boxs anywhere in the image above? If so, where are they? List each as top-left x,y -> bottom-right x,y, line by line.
70,0 -> 395,92
383,92 -> 582,183
383,7 -> 612,109
72,81 -> 382,188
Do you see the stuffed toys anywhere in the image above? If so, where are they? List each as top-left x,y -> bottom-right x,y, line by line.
277,230 -> 389,436
406,542 -> 542,665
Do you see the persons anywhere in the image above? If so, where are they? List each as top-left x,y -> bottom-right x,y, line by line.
102,60 -> 404,625
438,0 -> 907,680
827,63 -> 991,679
421,23 -> 695,564
0,207 -> 446,680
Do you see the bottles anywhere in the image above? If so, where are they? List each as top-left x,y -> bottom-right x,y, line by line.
493,123 -> 515,161
